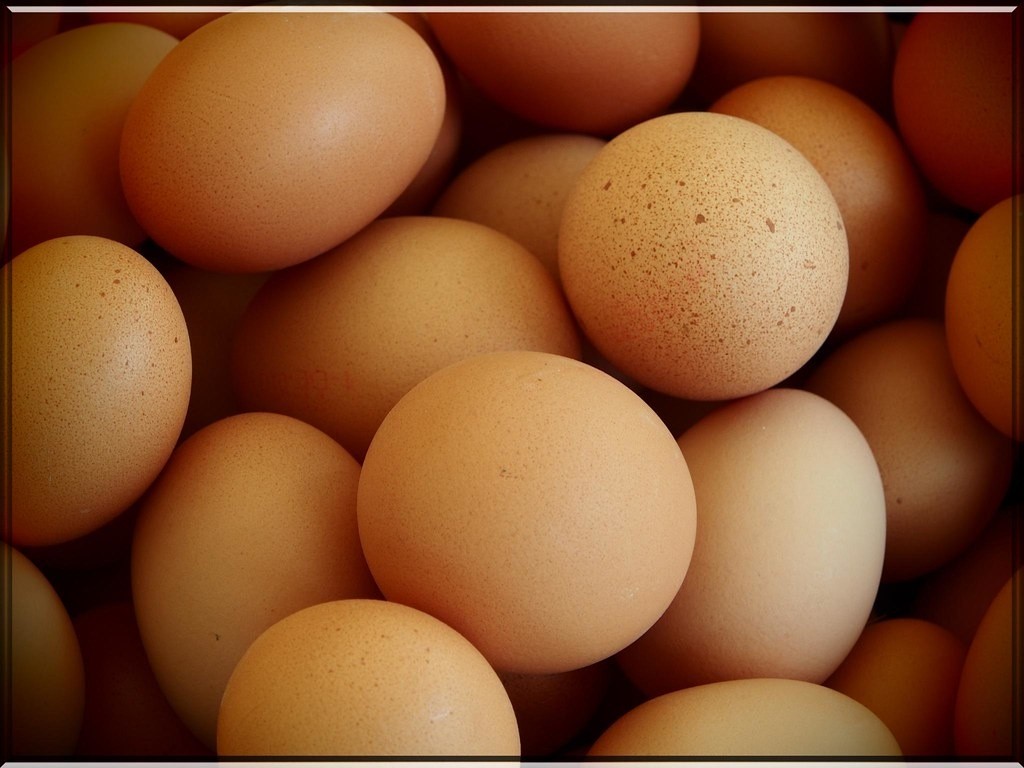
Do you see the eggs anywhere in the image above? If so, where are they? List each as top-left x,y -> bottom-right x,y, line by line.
9,11 -> 1014,754
